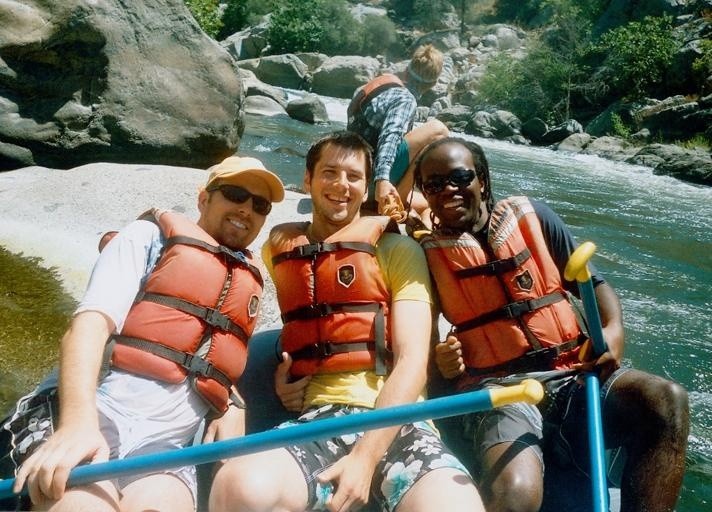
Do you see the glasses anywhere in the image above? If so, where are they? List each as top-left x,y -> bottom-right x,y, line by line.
205,184 -> 272,215
421,168 -> 480,196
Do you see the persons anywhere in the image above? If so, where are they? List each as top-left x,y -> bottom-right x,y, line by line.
413,135 -> 689,511
10,155 -> 286,511
207,131 -> 488,510
347,44 -> 451,243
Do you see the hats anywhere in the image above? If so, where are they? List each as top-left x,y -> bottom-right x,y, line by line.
204,156 -> 285,202
